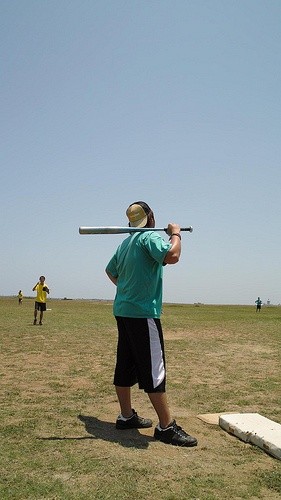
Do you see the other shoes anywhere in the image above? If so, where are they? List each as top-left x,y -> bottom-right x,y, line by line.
34,319 -> 42,325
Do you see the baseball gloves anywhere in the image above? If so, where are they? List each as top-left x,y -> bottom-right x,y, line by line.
42,286 -> 48,292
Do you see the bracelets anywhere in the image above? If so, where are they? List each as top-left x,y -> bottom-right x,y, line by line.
168,232 -> 181,240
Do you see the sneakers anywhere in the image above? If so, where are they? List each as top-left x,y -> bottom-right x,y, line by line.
116,409 -> 152,429
154,420 -> 197,446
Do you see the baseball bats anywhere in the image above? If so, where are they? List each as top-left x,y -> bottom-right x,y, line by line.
78,226 -> 193,234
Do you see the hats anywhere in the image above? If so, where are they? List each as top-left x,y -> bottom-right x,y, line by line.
126,201 -> 151,228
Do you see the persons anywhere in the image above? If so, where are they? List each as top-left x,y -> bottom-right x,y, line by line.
255,297 -> 262,312
105,202 -> 197,447
33,276 -> 50,325
17,291 -> 23,305
